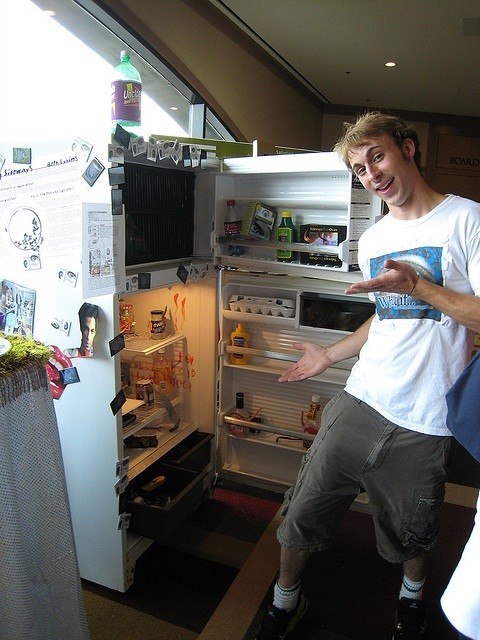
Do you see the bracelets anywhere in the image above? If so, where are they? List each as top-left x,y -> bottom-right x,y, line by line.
408,276 -> 421,296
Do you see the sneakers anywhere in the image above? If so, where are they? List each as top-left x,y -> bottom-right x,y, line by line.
390,595 -> 427,640
255,592 -> 308,639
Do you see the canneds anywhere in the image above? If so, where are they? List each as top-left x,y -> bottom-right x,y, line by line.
121,304 -> 133,334
137,379 -> 154,410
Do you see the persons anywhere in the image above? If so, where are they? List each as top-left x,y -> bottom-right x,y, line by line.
436,349 -> 478,638
0,281 -> 15,331
259,113 -> 478,639
63,302 -> 97,358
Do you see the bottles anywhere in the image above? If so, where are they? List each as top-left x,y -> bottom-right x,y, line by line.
153,348 -> 172,406
229,392 -> 249,438
111,50 -> 142,139
303,395 -> 321,449
275,212 -> 298,263
229,323 -> 250,365
120,304 -> 135,341
224,199 -> 246,256
